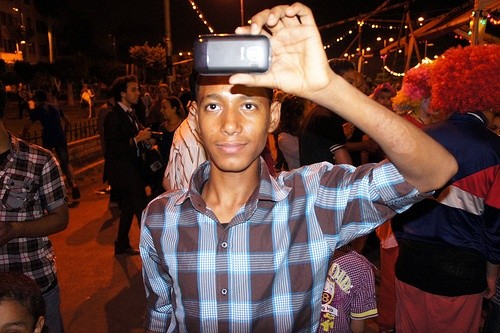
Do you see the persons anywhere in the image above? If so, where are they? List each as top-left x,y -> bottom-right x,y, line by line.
316,247 -> 379,333
23,91 -> 80,209
0,273 -> 45,333
0,90 -> 69,333
376,45 -> 500,333
103,75 -> 154,255
99,83 -> 192,193
81,84 -> 95,118
262,58 -> 397,173
139,2 -> 459,333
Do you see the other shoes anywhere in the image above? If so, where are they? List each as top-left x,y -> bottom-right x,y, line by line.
72,185 -> 80,199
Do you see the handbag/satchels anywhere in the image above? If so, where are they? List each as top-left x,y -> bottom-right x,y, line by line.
145,144 -> 163,177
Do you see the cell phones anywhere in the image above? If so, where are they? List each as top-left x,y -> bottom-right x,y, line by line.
193,33 -> 269,73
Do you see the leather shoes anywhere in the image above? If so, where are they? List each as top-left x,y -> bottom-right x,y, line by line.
116,248 -> 140,255
